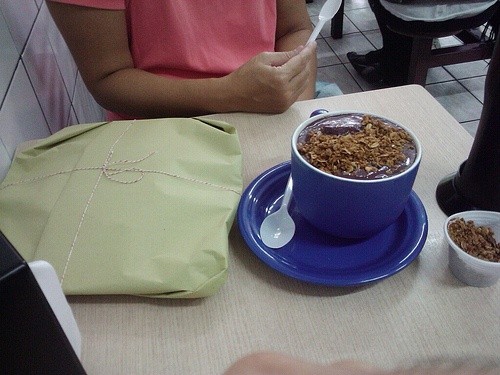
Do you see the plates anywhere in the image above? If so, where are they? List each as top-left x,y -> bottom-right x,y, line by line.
237,160 -> 428,286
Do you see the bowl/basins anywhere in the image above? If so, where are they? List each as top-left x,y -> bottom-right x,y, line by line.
445,210 -> 500,287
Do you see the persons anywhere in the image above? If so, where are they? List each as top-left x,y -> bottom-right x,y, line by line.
44,0 -> 318,120
347,0 -> 500,84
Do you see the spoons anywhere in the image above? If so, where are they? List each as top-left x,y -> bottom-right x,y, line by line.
260,172 -> 295,248
305,0 -> 342,47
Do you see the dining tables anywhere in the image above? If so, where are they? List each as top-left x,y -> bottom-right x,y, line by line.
66,83 -> 499,375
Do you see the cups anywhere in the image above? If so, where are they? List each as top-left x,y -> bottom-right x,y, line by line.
291,108 -> 422,237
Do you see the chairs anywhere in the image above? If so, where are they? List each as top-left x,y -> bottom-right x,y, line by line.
367,0 -> 500,87
